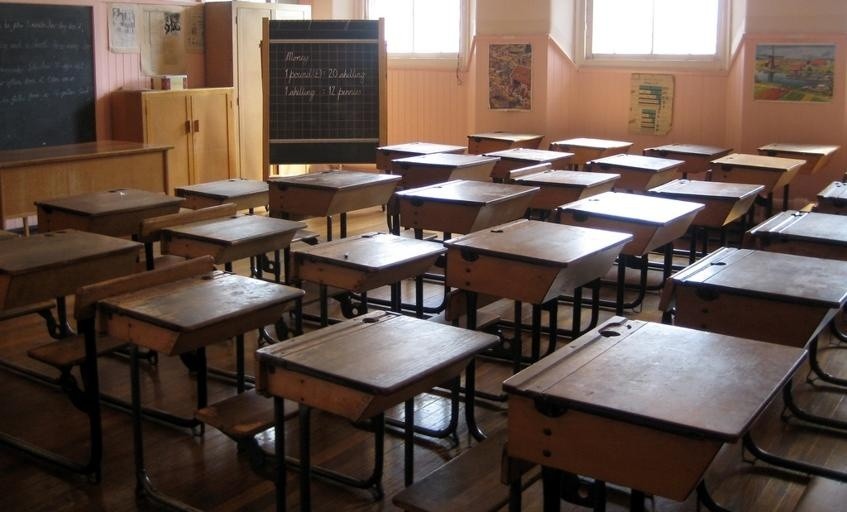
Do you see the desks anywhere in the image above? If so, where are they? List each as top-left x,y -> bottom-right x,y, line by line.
0,128 -> 846,512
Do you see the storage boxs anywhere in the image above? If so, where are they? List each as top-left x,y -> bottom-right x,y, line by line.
149,73 -> 188,93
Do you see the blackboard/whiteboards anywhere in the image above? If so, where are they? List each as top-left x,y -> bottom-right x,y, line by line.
0,0 -> 97,151
261,18 -> 387,164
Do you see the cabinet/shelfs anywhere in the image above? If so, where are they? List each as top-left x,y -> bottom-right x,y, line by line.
108,85 -> 242,213
200,2 -> 314,214
